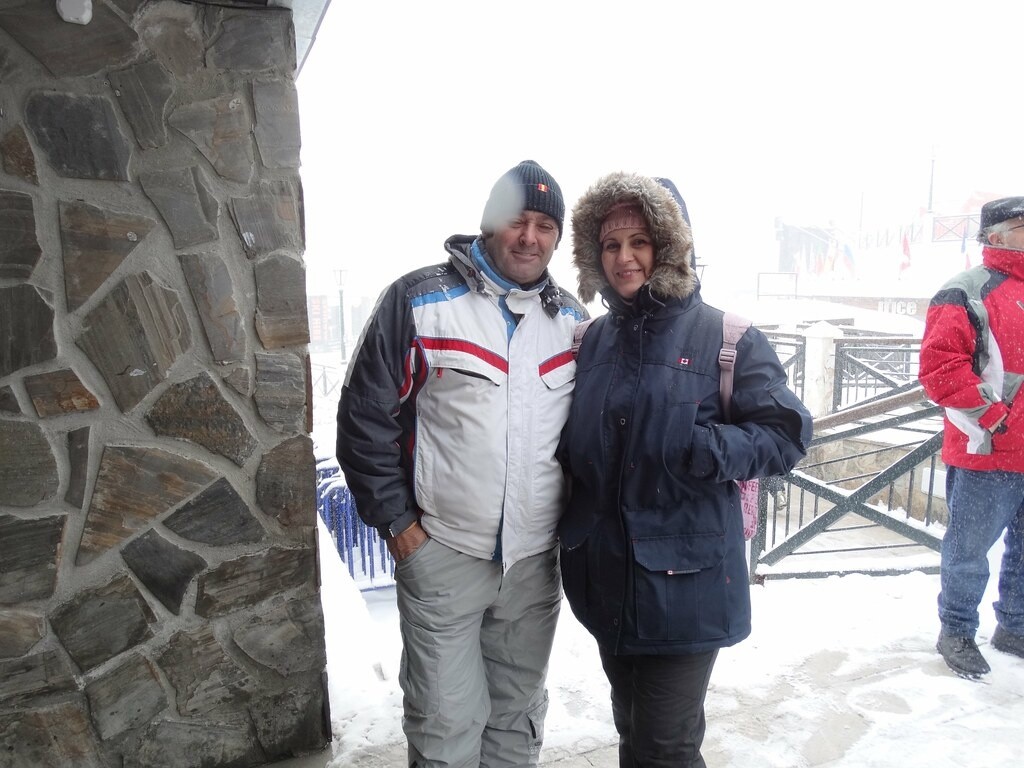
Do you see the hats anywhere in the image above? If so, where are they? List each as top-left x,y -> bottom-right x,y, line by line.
599,205 -> 648,243
480,160 -> 565,238
980,196 -> 1024,228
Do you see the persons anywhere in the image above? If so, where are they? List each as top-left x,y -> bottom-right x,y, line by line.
919,196 -> 1024,683
553,169 -> 816,768
336,159 -> 591,768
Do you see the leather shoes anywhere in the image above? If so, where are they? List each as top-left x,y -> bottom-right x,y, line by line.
991,625 -> 1024,658
935,629 -> 990,678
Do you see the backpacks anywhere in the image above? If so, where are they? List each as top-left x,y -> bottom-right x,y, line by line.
568,310 -> 760,540
